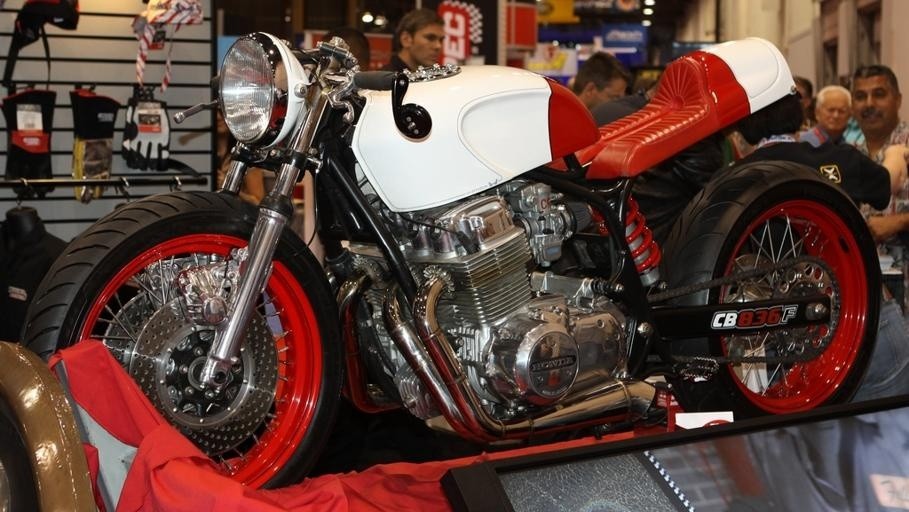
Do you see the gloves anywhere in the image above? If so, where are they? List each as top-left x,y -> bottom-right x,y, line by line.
2,91 -> 58,198
68,89 -> 117,204
124,100 -> 172,171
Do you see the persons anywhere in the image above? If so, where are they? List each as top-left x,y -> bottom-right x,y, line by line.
789,65 -> 907,311
321,28 -> 371,71
571,51 -> 661,127
217,106 -> 265,205
376,9 -> 446,73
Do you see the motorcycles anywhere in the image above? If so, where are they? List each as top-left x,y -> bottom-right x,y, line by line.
21,30 -> 892,491
